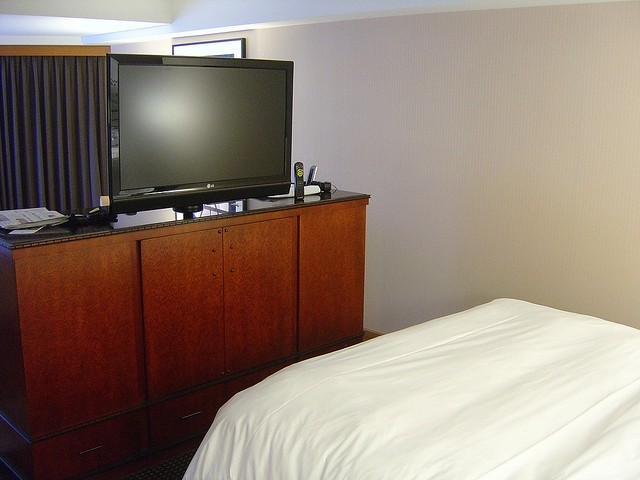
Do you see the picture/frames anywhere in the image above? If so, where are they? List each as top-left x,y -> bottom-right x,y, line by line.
172,38 -> 246,58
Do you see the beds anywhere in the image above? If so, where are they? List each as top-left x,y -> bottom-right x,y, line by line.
178,295 -> 638,480
0,185 -> 370,480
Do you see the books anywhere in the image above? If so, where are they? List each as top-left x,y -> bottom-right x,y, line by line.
0,207 -> 67,230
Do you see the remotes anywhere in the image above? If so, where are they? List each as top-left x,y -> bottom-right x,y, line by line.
294,162 -> 304,200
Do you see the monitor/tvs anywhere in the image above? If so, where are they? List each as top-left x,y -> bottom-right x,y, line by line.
106,53 -> 294,213
